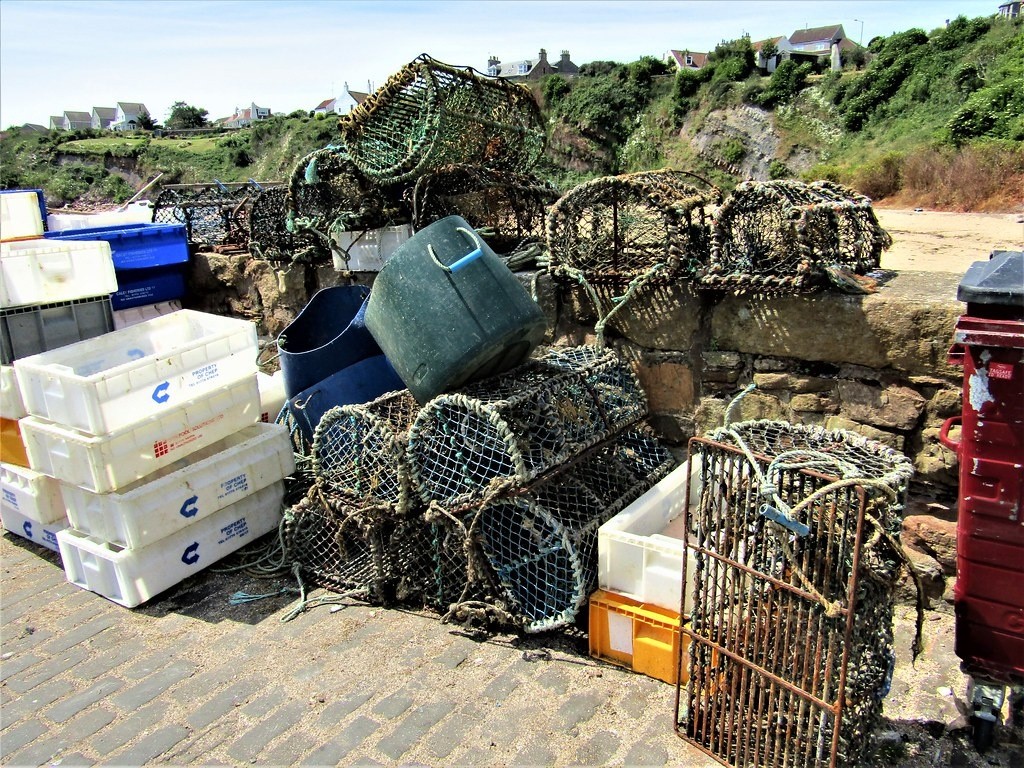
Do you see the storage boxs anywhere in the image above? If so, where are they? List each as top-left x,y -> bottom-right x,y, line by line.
592,450 -> 789,698
331,223 -> 415,273
0,187 -> 295,612
